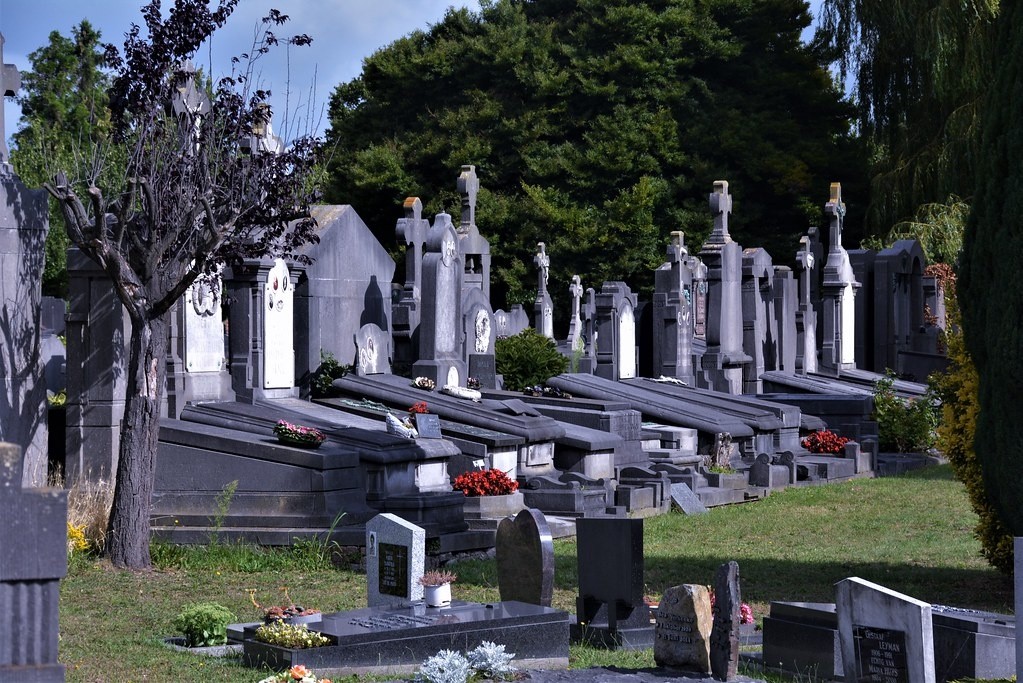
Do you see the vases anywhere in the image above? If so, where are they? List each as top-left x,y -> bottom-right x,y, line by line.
424,582 -> 452,608
271,613 -> 322,624
279,438 -> 321,449
524,392 -> 542,396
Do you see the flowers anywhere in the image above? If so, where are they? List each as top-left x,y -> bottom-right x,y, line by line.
408,402 -> 429,422
707,585 -> 754,624
411,376 -> 435,391
448,468 -> 519,497
544,386 -> 572,398
801,429 -> 864,457
244,586 -> 320,624
415,569 -> 458,586
467,377 -> 484,390
273,419 -> 327,443
524,386 -> 543,397
